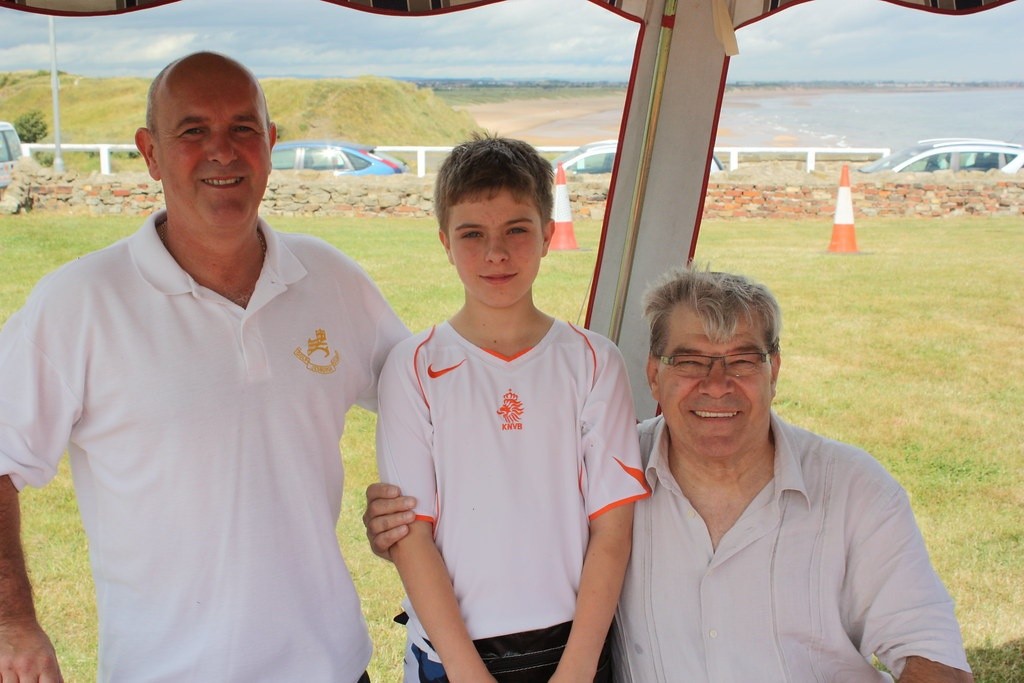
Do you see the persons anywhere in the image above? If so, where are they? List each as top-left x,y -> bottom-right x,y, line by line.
362,270 -> 975,683
0,50 -> 416,683
374,138 -> 653,683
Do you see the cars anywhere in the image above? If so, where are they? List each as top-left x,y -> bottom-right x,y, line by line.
271,139 -> 412,175
0,122 -> 24,187
550,139 -> 724,185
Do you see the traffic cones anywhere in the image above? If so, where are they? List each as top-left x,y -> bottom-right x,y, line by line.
826,165 -> 860,253
548,162 -> 578,250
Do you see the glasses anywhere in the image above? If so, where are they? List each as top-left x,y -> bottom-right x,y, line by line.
650,336 -> 779,377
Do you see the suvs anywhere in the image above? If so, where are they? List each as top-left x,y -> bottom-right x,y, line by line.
858,137 -> 1024,173
